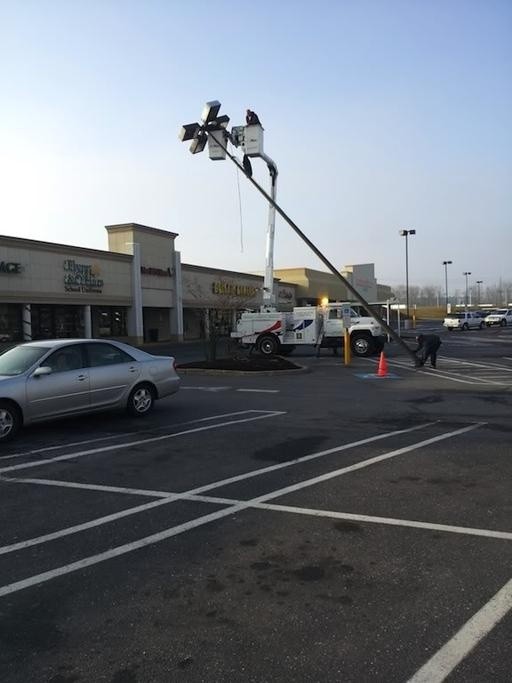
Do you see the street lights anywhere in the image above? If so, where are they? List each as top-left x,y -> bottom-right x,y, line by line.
443,261 -> 452,314
464,272 -> 471,312
476,281 -> 483,304
400,230 -> 415,319
178,101 -> 424,367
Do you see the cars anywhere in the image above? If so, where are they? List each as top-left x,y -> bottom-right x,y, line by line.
443,308 -> 512,331
0,337 -> 180,442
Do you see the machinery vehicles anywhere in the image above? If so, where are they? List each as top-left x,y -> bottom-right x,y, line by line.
208,124 -> 384,357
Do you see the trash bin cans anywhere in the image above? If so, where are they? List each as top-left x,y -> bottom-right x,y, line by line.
149,329 -> 158,342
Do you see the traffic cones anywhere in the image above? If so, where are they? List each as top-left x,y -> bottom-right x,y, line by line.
378,352 -> 387,377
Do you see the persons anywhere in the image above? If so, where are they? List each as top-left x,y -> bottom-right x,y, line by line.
412,333 -> 443,368
